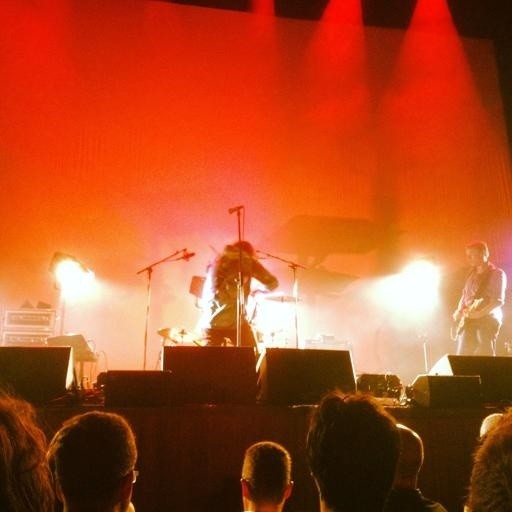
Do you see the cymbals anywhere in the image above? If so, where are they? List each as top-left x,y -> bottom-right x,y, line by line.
158,326 -> 197,343
266,294 -> 301,303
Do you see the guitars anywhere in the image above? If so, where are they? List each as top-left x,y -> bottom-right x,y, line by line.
450,295 -> 488,340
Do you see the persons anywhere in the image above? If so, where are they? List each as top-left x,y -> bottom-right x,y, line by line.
0,390 -> 57,512
464,399 -> 512,512
479,413 -> 503,443
304,387 -> 405,512
239,440 -> 295,512
200,240 -> 279,365
451,239 -> 508,356
47,409 -> 139,512
384,422 -> 449,512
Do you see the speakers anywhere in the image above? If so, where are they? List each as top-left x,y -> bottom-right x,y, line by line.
0,347 -> 77,403
356,374 -> 401,401
105,370 -> 178,407
409,376 -> 480,408
427,355 -> 512,403
256,348 -> 356,403
163,346 -> 257,405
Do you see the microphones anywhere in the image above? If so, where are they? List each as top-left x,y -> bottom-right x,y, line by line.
228,205 -> 243,214
181,252 -> 194,259
